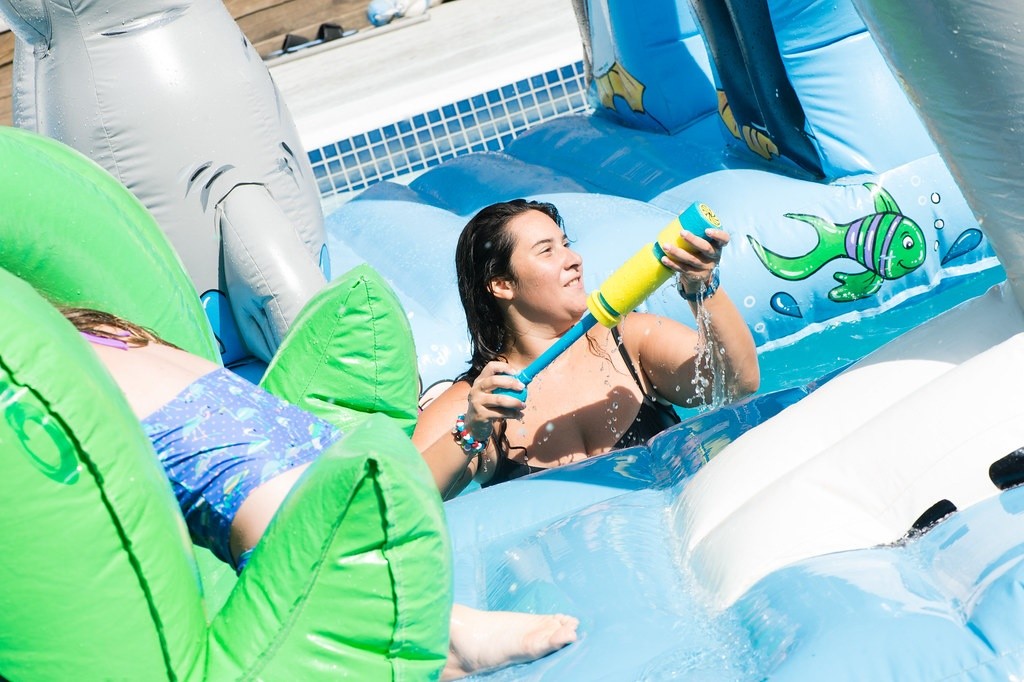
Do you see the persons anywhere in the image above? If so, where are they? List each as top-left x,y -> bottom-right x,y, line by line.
410,200 -> 759,502
57,306 -> 581,681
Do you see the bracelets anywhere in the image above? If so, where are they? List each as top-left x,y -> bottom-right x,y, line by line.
451,415 -> 491,472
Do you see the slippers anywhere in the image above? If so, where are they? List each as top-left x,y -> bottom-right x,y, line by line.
287,23 -> 358,51
266,34 -> 311,56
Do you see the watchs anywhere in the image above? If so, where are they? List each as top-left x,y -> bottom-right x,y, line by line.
676,269 -> 720,301
